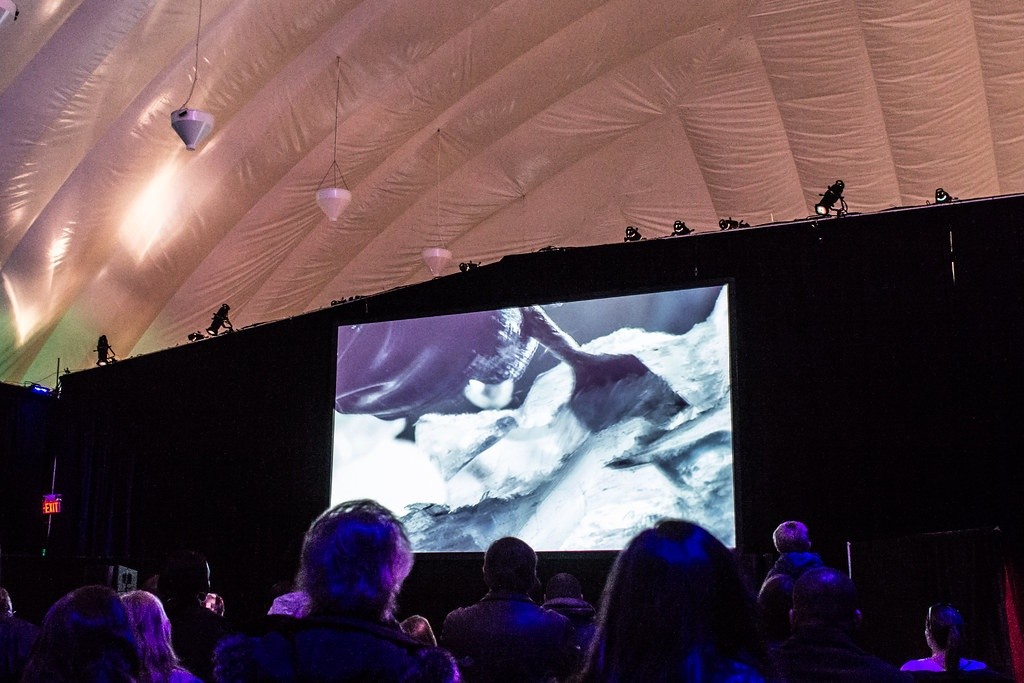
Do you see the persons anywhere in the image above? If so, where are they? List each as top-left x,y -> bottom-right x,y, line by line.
757,521 -> 909,683
441,537 -> 581,683
900,602 -> 988,672
541,573 -> 598,667
210,500 -> 463,683
0,585 -> 207,683
581,521 -> 783,683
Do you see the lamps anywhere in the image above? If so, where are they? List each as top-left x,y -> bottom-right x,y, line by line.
422,130 -> 452,277
818,180 -> 849,218
313,55 -> 352,221
206,303 -> 232,337
170,2 -> 228,154
96,335 -> 115,366
